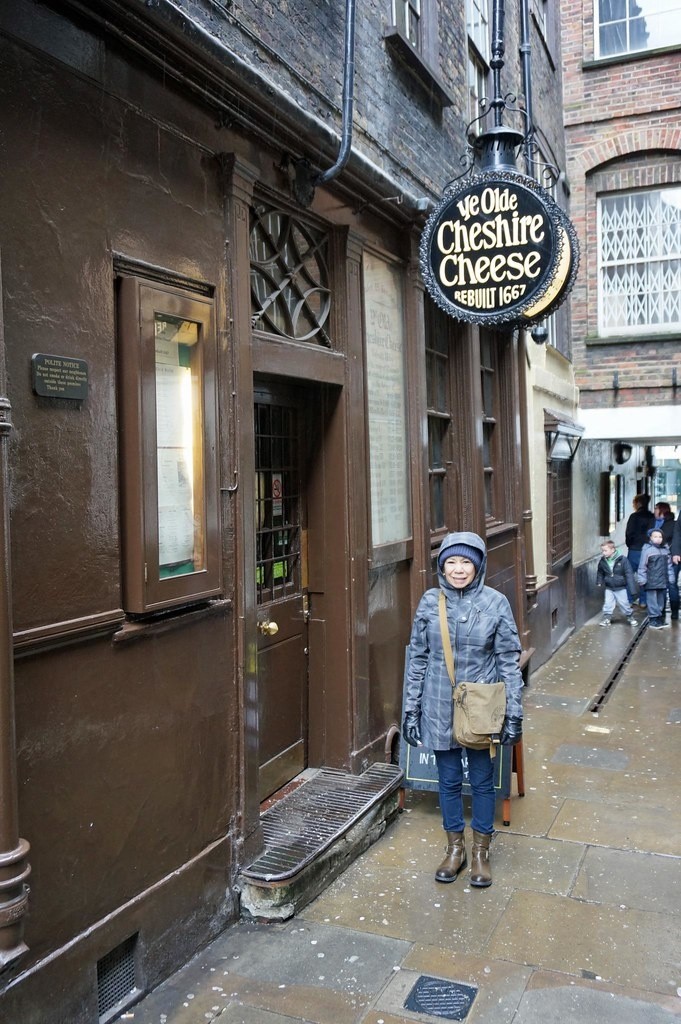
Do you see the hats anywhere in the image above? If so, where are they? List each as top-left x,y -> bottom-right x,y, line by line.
635,493 -> 651,506
647,528 -> 664,537
439,543 -> 482,573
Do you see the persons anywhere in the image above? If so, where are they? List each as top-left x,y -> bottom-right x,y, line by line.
637,527 -> 676,630
671,511 -> 681,610
403,531 -> 526,889
596,540 -> 639,628
644,500 -> 679,620
624,492 -> 656,609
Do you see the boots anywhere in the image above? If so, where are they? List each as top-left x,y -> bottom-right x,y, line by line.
670,600 -> 679,619
469,829 -> 493,886
435,830 -> 468,882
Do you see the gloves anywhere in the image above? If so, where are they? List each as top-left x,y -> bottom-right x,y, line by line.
402,710 -> 422,747
500,714 -> 522,745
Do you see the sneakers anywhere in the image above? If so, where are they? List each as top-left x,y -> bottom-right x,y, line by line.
598,618 -> 611,626
628,618 -> 638,626
649,618 -> 669,629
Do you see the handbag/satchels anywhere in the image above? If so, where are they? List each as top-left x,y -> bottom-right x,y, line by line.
451,680 -> 506,763
